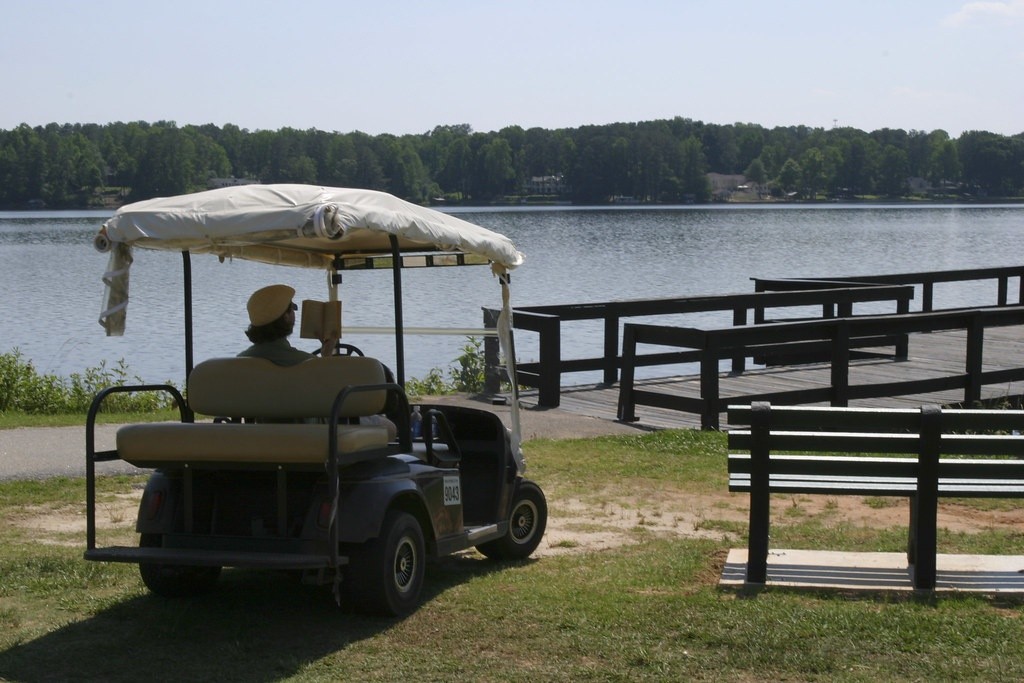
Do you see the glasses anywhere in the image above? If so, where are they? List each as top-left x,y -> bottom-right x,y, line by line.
288,303 -> 298,311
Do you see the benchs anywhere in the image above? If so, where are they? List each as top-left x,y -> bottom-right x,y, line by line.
117,358 -> 390,470
725,404 -> 1024,592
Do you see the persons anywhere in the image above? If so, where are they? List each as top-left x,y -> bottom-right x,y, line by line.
237,285 -> 397,442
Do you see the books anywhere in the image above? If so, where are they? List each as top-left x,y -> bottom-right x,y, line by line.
300,300 -> 341,339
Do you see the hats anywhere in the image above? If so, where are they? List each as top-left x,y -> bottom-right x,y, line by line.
248,285 -> 296,326
